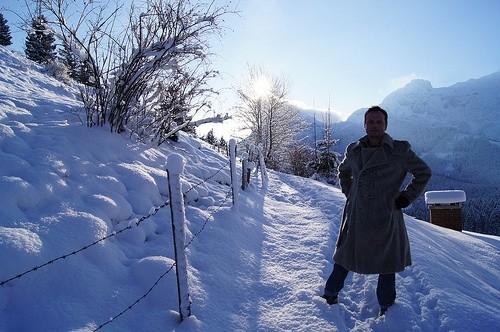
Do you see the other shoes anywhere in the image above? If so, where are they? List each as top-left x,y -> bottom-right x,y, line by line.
377,307 -> 387,319
320,294 -> 339,305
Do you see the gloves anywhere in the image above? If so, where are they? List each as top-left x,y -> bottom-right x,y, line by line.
395,195 -> 410,210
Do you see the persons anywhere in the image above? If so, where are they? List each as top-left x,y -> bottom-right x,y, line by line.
320,106 -> 432,317
